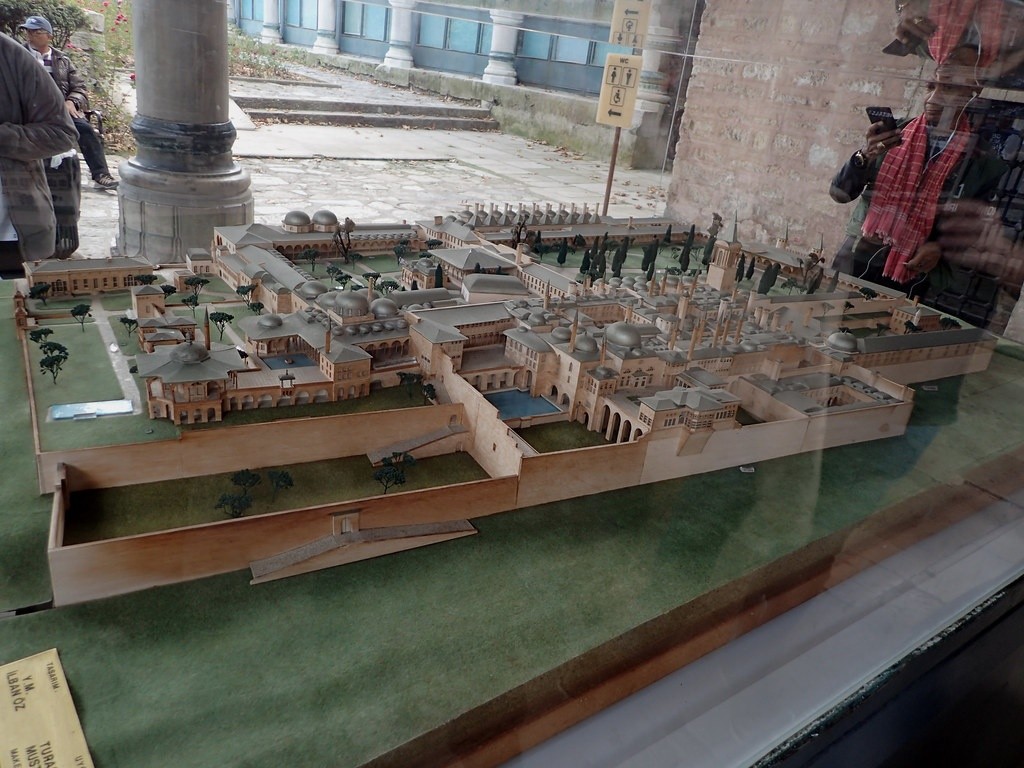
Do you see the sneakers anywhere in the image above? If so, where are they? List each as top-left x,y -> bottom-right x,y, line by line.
95,174 -> 120,190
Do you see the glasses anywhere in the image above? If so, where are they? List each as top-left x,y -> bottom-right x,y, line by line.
26,31 -> 49,37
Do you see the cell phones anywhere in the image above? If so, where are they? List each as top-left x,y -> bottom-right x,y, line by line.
882,16 -> 937,58
866,107 -> 902,147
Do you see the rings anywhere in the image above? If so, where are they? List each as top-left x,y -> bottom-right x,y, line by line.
912,17 -> 924,24
917,264 -> 922,268
877,142 -> 885,151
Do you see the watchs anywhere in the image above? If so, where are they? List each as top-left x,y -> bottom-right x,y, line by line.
897,0 -> 911,14
853,150 -> 871,169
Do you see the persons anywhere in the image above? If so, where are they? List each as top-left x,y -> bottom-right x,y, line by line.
0,16 -> 120,280
819,63 -> 988,306
893,0 -> 1024,422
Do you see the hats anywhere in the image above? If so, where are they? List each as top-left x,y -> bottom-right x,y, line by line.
18,16 -> 53,34
